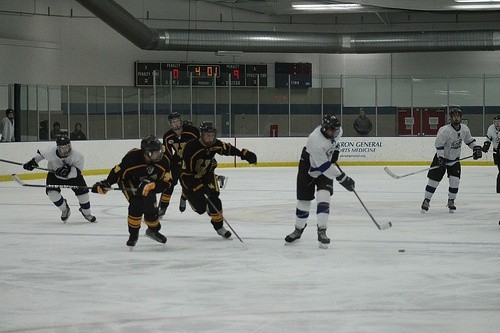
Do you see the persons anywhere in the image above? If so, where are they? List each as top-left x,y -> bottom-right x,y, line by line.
0,109 -> 16,143
353,107 -> 372,137
159,112 -> 228,219
483,115 -> 500,226
181,123 -> 257,242
91,134 -> 173,247
39,120 -> 69,140
24,136 -> 96,223
284,114 -> 355,248
421,109 -> 482,214
70,123 -> 88,141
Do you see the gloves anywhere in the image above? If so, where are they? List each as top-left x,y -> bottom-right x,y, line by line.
331,150 -> 340,164
92,181 -> 111,195
184,178 -> 195,189
23,158 -> 39,170
473,148 -> 482,159
438,158 -> 447,171
154,181 -> 165,193
241,149 -> 256,164
482,141 -> 491,153
56,165 -> 70,177
336,174 -> 355,192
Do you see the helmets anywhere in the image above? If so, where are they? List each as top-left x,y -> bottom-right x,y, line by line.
55,135 -> 69,147
493,114 -> 500,121
321,114 -> 340,130
449,108 -> 463,115
141,112 -> 215,155
6,109 -> 13,114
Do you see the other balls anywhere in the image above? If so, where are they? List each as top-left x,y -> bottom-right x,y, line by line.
399,250 -> 405,252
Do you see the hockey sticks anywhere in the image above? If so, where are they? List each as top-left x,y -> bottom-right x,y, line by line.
488,149 -> 497,154
335,162 -> 393,230
0,158 -> 56,173
205,193 -> 248,250
384,154 -> 475,179
12,173 -> 137,191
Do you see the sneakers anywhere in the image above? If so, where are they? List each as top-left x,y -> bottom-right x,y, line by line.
80,207 -> 97,223
218,175 -> 228,188
316,223 -> 330,248
179,196 -> 186,213
146,229 -> 166,244
216,228 -> 233,240
158,208 -> 167,218
447,199 -> 456,213
126,234 -> 138,251
60,200 -> 70,222
285,222 -> 307,246
421,198 -> 430,213
